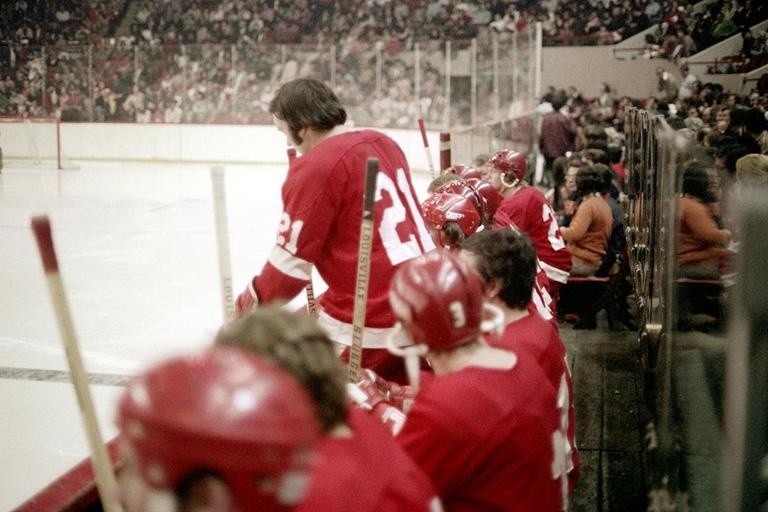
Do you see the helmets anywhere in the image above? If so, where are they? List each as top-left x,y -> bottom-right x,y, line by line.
416,148 -> 524,244
111,343 -> 320,512
386,247 -> 484,356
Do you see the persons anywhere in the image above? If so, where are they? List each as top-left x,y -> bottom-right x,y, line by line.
1,1 -> 417,133
417,1 -> 767,338
346,247 -> 576,511
231,77 -> 445,396
101,340 -> 446,512
452,224 -> 581,511
209,302 -> 445,511
